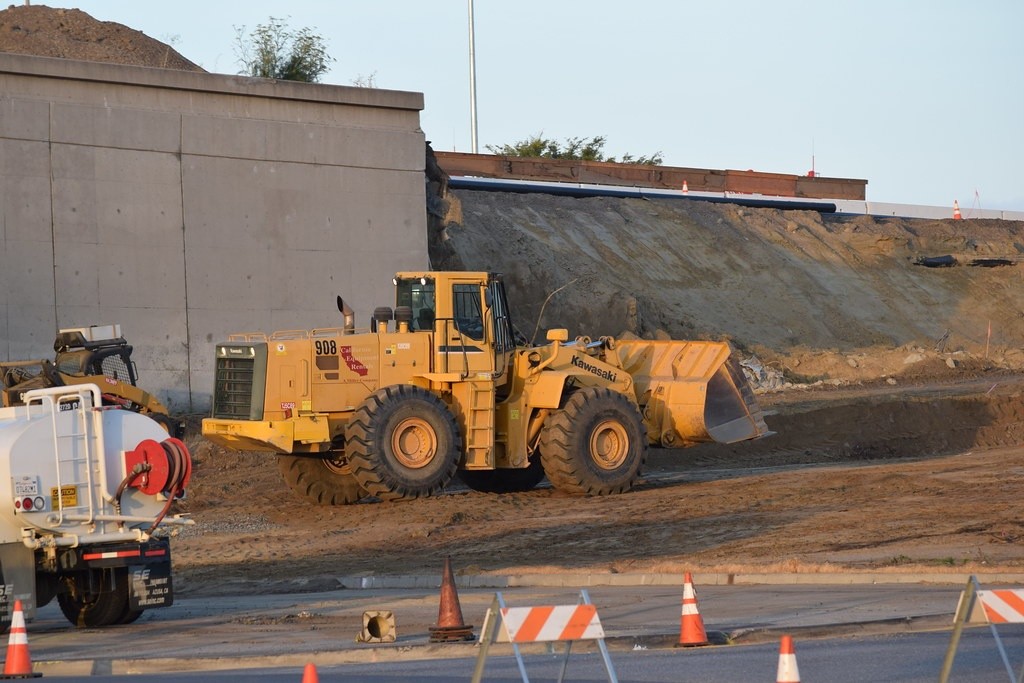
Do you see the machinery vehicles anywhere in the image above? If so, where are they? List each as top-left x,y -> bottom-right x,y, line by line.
0,324 -> 188,441
0,381 -> 193,634
201,270 -> 777,507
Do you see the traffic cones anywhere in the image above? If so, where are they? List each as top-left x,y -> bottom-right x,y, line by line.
355,610 -> 396,643
954,200 -> 961,219
301,663 -> 319,683
429,557 -> 475,642
683,180 -> 689,192
0,599 -> 43,679
675,572 -> 709,648
775,635 -> 802,683
